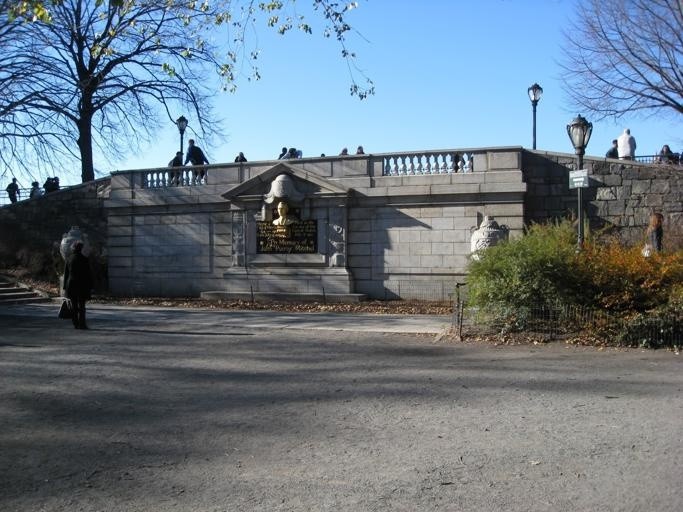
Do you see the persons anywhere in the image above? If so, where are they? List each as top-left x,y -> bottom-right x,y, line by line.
28,181 -> 42,200
606,139 -> 619,160
61,241 -> 94,330
645,213 -> 665,254
43,176 -> 60,195
320,153 -> 325,158
356,146 -> 365,154
339,148 -> 351,155
184,139 -> 209,178
277,146 -> 299,160
7,178 -> 21,203
167,152 -> 183,186
656,145 -> 678,164
272,202 -> 296,226
234,151 -> 247,162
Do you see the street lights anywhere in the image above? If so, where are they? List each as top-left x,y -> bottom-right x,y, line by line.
528,84 -> 543,150
566,113 -> 592,245
176,115 -> 188,166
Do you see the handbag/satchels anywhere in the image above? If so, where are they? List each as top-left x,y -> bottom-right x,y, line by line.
58,300 -> 74,320
641,243 -> 652,257
